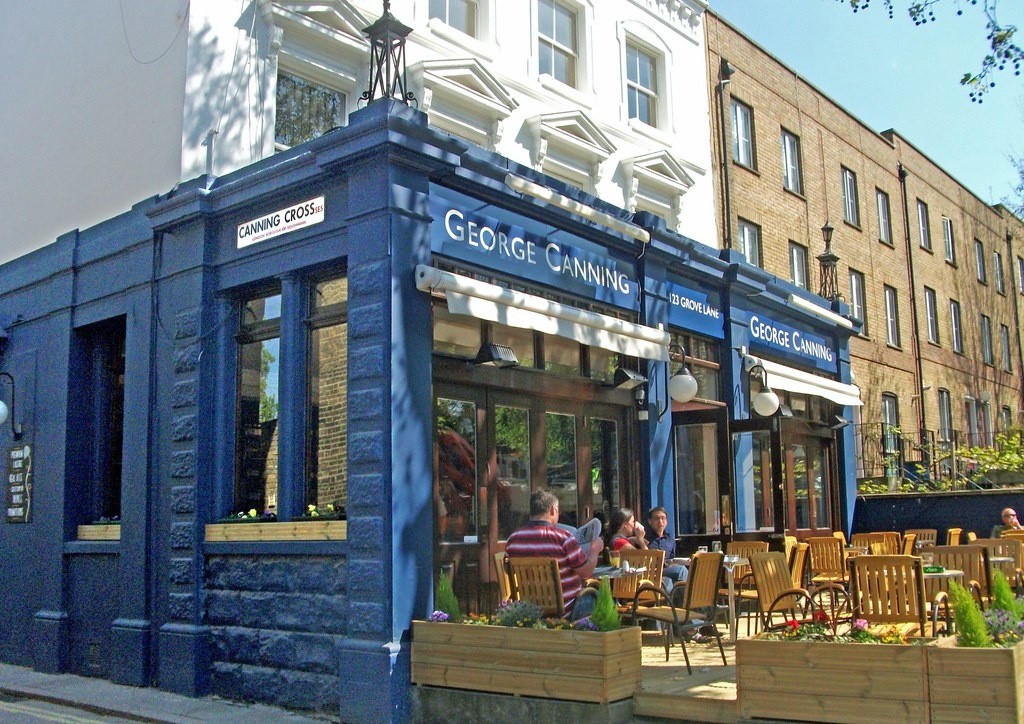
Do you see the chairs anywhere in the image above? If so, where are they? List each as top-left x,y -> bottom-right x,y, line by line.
490,528 -> 1024,641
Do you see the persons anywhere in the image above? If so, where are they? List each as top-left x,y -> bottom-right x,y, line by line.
990,507 -> 1023,555
603,508 -> 723,643
439,476 -> 471,518
641,506 -> 729,615
505,487 -> 604,621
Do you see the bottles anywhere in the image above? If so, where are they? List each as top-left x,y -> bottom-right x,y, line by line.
603,544 -> 610,566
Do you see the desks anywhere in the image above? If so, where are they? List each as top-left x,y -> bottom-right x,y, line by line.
667,557 -> 750,643
914,541 -> 935,553
867,567 -> 965,589
587,565 -> 647,627
980,555 -> 1014,563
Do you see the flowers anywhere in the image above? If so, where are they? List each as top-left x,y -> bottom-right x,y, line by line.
753,608 -> 906,646
427,568 -> 620,632
97,514 -> 120,522
300,503 -> 342,516
227,508 -> 273,518
944,566 -> 1024,649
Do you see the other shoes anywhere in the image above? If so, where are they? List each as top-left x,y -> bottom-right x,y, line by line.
700,625 -> 723,636
684,629 -> 712,644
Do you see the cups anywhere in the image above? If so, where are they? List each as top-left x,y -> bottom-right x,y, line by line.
922,553 -> 933,567
712,541 -> 721,553
610,551 -> 620,567
635,521 -> 645,531
1009,518 -> 1016,526
698,546 -> 708,554
731,555 -> 739,562
724,555 -> 731,562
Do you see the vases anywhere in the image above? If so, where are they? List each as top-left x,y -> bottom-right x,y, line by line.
733,629 -> 931,724
409,619 -> 642,704
291,513 -> 345,521
218,517 -> 276,522
926,637 -> 1024,724
92,520 -> 122,527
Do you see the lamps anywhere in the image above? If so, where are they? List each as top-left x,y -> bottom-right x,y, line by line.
657,342 -> 699,420
822,219 -> 836,252
772,404 -> 795,421
461,343 -> 520,370
826,415 -> 850,430
600,367 -> 648,390
506,173 -> 651,258
719,57 -> 735,78
732,364 -> 779,439
784,293 -> 853,331
0,372 -> 23,440
899,165 -> 907,178
